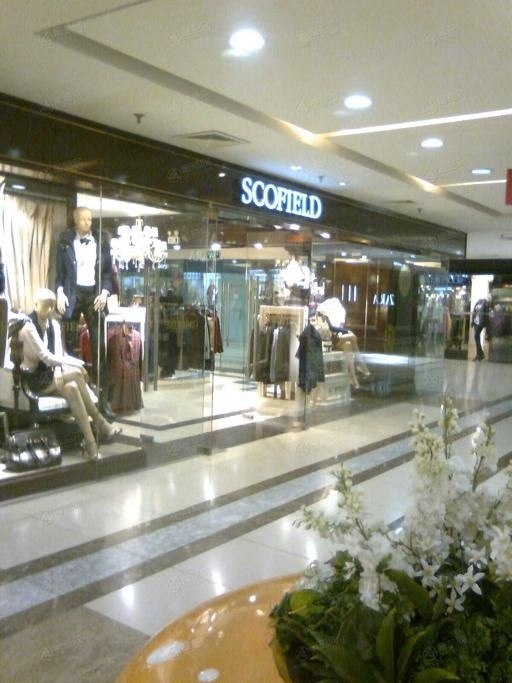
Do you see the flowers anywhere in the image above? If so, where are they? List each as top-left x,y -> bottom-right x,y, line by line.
269,391 -> 511,681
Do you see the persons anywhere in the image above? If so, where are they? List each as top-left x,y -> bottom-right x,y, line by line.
321,314 -> 369,390
450,287 -> 468,349
471,298 -> 489,361
207,280 -> 217,306
54,206 -> 114,389
19,287 -> 121,461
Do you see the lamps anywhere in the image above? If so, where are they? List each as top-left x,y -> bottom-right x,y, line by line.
109,218 -> 168,273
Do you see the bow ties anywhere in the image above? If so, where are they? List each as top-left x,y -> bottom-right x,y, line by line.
80,238 -> 90,244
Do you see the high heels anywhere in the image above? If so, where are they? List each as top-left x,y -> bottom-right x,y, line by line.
350,379 -> 360,389
357,366 -> 371,376
81,440 -> 102,461
93,419 -> 121,438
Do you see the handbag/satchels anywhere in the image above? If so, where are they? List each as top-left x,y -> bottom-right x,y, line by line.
6,427 -> 62,471
